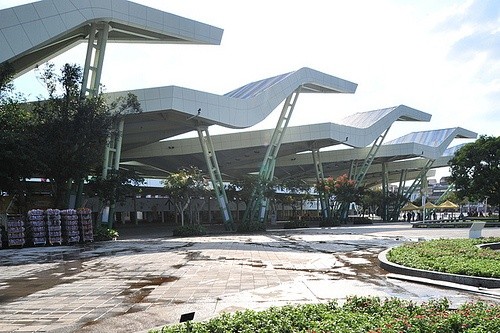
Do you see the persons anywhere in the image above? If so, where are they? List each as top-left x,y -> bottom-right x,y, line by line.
403,214 -> 406,221
488,210 -> 492,216
411,211 -> 415,222
467,210 -> 485,216
407,212 -> 412,221
460,213 -> 464,217
416,211 -> 420,221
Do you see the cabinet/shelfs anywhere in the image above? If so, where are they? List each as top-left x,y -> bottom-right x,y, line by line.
28,209 -> 45,246
78,208 -> 94,244
46,208 -> 62,245
7,213 -> 26,249
62,209 -> 80,245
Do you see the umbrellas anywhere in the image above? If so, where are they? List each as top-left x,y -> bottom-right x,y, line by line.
419,202 -> 437,209
400,203 -> 419,210
437,200 -> 458,208
